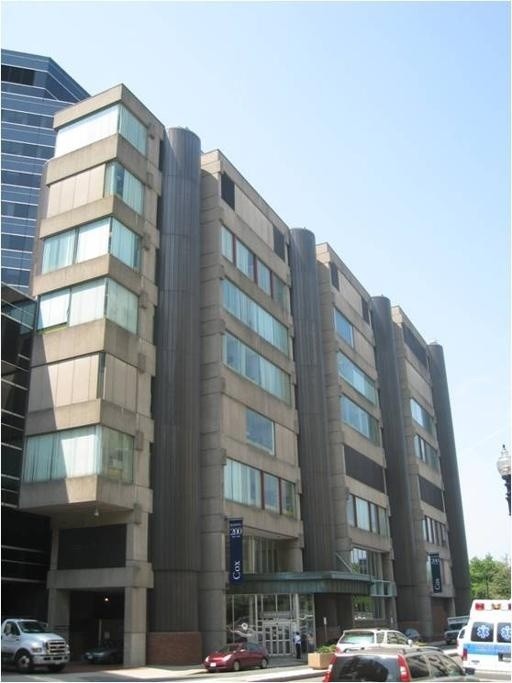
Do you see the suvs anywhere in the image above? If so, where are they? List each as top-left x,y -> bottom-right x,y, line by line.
324,644 -> 480,682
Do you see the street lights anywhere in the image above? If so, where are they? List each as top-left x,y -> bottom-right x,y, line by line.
497,445 -> 510,513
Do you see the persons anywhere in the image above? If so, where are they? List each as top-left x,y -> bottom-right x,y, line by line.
295,632 -> 301,659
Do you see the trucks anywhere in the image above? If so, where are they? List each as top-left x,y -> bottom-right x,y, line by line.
444,615 -> 470,643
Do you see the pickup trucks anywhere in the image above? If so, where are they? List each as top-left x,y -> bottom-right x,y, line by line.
1,618 -> 71,674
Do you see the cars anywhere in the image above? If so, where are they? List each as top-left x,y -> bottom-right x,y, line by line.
84,640 -> 123,664
335,627 -> 443,656
203,642 -> 268,671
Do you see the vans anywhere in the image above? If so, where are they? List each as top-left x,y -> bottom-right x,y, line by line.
455,599 -> 511,680
